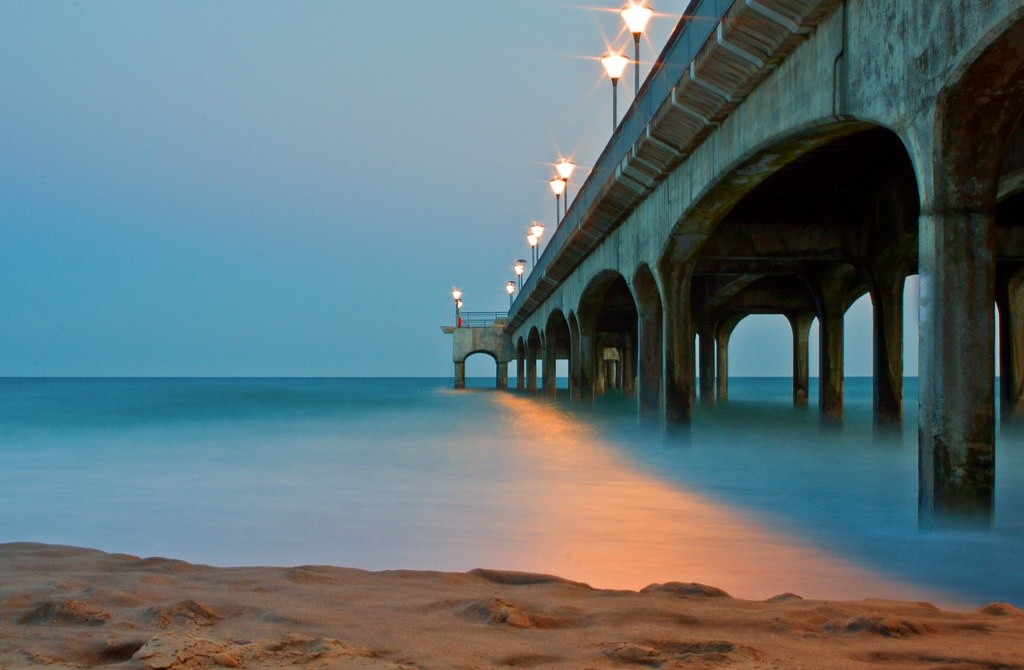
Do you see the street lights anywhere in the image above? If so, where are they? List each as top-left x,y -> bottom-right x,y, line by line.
506,282 -> 515,307
549,177 -> 566,228
455,301 -> 463,326
514,264 -> 524,293
452,290 -> 462,327
554,159 -> 575,220
526,234 -> 537,270
600,50 -> 629,131
530,224 -> 544,263
620,4 -> 655,98
